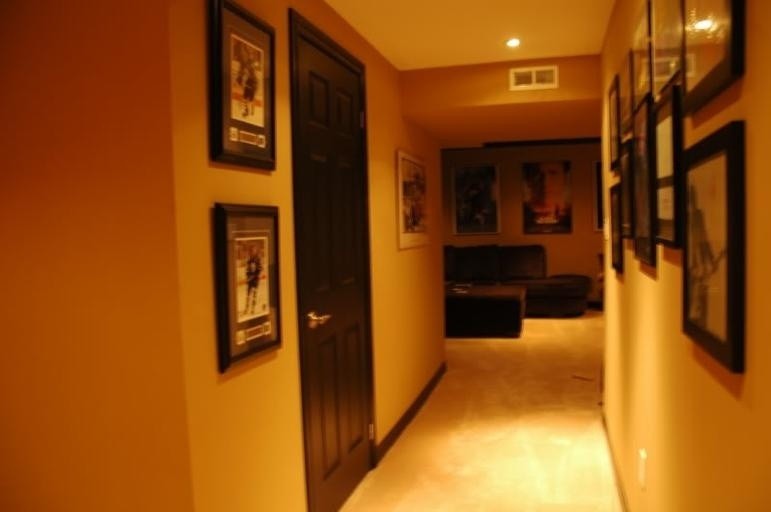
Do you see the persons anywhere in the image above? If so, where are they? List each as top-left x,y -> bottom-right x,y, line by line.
689,184 -> 721,329
521,161 -> 573,235
242,243 -> 263,316
236,43 -> 258,116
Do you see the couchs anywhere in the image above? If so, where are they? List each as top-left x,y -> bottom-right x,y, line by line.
445,244 -> 547,285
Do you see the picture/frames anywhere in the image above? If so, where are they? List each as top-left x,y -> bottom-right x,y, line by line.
209,0 -> 276,173
395,148 -> 432,250
609,182 -> 624,274
450,164 -> 503,236
607,74 -> 619,169
632,7 -> 651,111
647,2 -> 687,109
619,140 -> 633,240
678,119 -> 744,374
654,181 -> 680,249
631,92 -> 656,268
651,82 -> 683,184
214,201 -> 282,373
619,49 -> 636,137
593,160 -> 604,233
679,2 -> 745,114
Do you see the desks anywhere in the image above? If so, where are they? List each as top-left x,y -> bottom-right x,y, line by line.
444,284 -> 527,339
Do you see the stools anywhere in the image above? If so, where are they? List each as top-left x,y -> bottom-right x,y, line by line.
510,274 -> 593,319
